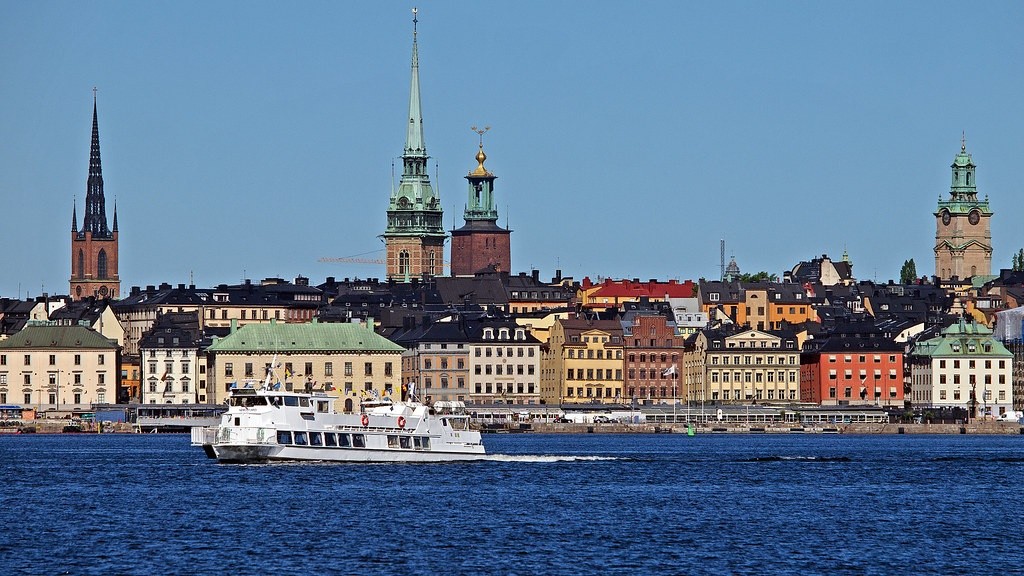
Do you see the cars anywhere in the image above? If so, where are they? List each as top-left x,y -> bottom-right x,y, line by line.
553,418 -> 573,423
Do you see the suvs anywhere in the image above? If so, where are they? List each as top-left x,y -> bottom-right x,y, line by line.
593,415 -> 614,423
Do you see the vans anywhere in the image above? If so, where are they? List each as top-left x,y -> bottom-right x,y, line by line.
996,410 -> 1024,422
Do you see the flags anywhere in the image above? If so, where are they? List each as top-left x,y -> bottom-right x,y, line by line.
230,380 -> 238,388
662,365 -> 674,375
273,368 -> 412,402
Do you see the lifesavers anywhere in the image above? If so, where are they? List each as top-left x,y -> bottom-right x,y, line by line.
361,414 -> 369,426
398,416 -> 406,427
256,427 -> 264,441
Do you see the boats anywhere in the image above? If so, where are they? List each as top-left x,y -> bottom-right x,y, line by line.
189,354 -> 487,465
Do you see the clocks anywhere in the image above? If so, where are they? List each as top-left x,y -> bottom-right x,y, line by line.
399,197 -> 409,209
942,210 -> 951,226
968,211 -> 980,225
